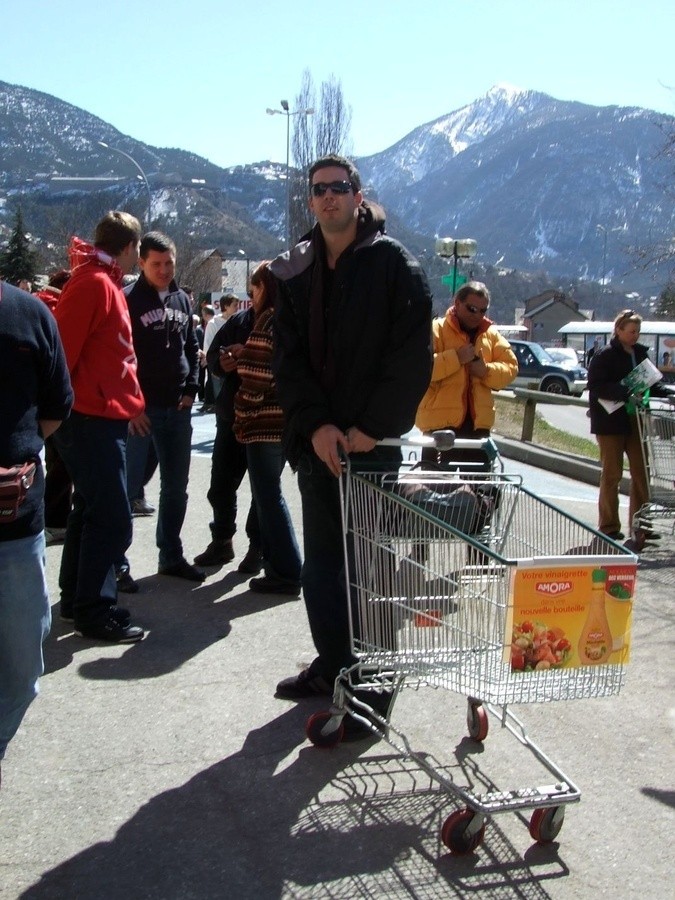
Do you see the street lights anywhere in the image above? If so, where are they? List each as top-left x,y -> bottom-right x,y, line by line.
266,100 -> 315,253
96,141 -> 152,232
434,237 -> 477,301
595,224 -> 622,322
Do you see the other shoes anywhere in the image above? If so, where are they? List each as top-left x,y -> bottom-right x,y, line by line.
630,531 -> 664,539
276,665 -> 346,698
606,531 -> 625,540
402,552 -> 424,567
72,621 -> 145,642
43,526 -> 69,544
208,405 -> 217,413
59,603 -> 131,621
157,560 -> 208,582
197,405 -> 209,413
249,576 -> 301,596
199,398 -> 206,402
482,563 -> 504,575
341,706 -> 387,739
129,500 -> 155,516
194,541 -> 234,564
116,570 -> 141,594
240,548 -> 264,575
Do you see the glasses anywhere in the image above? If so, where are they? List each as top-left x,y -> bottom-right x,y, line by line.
460,301 -> 488,314
311,180 -> 352,196
619,312 -> 634,327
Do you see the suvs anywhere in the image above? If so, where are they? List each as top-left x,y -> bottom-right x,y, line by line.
500,339 -> 589,398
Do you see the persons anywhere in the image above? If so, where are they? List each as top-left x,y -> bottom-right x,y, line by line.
663,351 -> 673,368
587,341 -> 601,366
0,210 -> 301,762
399,279 -> 519,565
586,308 -> 663,542
260,151 -> 433,745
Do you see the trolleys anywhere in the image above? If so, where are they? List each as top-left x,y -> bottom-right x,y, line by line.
306,427 -> 640,856
632,397 -> 675,553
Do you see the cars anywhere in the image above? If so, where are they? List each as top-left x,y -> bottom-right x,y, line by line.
544,348 -> 584,368
